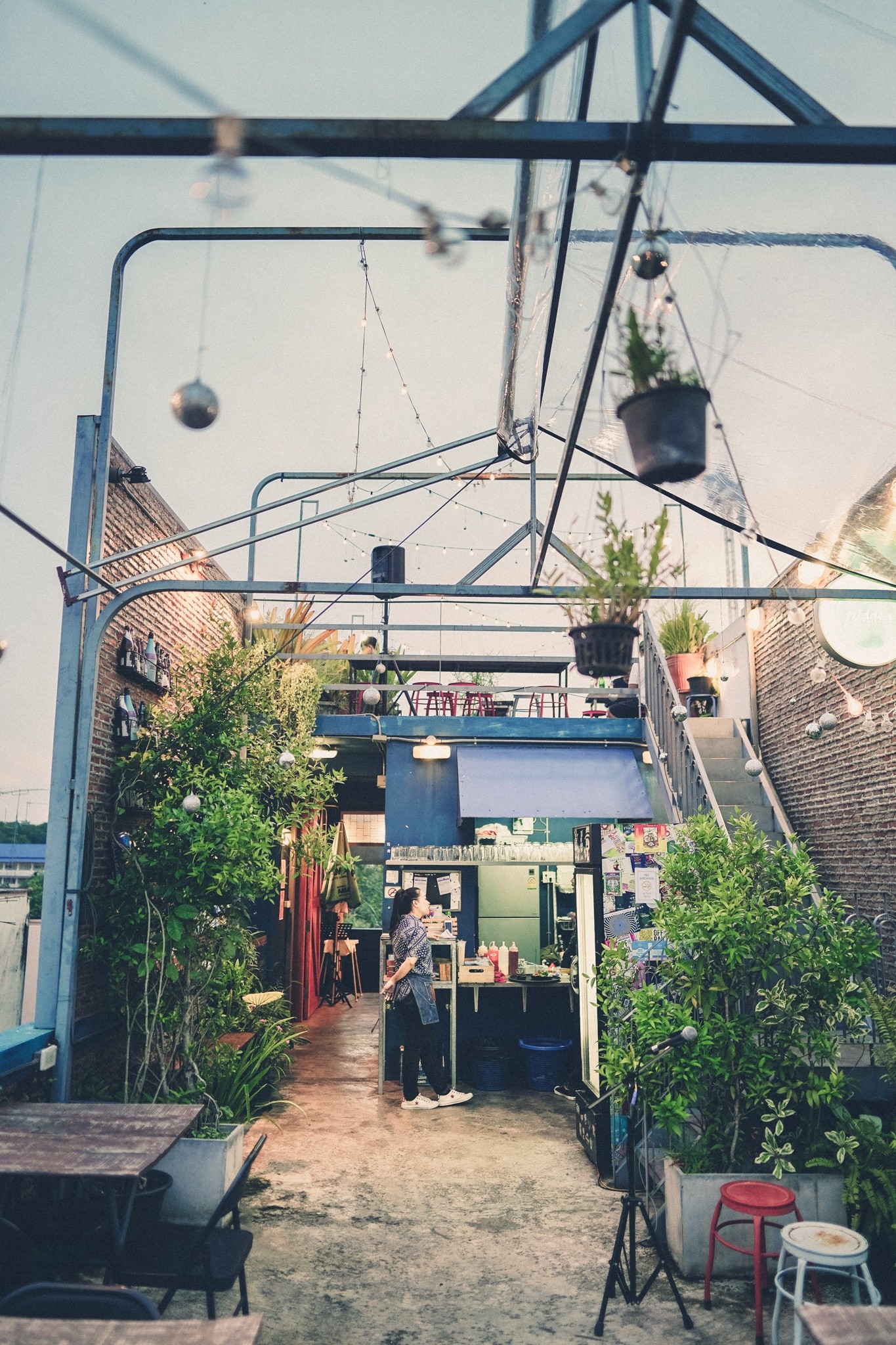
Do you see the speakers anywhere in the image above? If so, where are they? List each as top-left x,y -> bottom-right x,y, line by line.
371,545 -> 404,599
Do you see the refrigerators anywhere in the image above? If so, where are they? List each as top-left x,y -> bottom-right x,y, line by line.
478,865 -> 541,965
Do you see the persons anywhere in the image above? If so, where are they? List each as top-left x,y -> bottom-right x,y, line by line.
360,636 -> 377,654
379,887 -> 473,1110
604,641 -> 646,719
554,912 -> 582,1101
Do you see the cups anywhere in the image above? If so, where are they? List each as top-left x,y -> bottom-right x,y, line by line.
456,941 -> 466,966
390,842 -> 574,863
517,975 -> 526,980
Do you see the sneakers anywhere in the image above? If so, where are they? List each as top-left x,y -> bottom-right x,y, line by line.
401,1093 -> 439,1110
437,1090 -> 473,1106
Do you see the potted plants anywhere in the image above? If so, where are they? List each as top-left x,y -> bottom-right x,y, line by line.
658,598 -> 718,692
686,669 -> 713,695
82,599 -> 419,1123
533,487 -> 671,678
611,307 -> 709,481
582,802 -> 896,1277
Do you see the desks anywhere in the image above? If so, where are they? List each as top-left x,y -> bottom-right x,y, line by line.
318,938 -> 363,1001
0,1311 -> 266,1345
348,660 -> 570,714
0,1104 -> 204,1283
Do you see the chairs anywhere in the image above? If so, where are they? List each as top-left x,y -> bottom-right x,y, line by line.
0,1280 -> 165,1320
107,1132 -> 269,1319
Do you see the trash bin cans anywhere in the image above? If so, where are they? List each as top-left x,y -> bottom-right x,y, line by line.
519,1036 -> 573,1091
465,1036 -> 517,1091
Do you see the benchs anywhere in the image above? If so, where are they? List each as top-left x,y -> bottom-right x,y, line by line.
160,1031 -> 257,1071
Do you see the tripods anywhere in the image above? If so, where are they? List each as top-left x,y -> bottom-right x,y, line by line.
586,1045 -> 692,1336
360,598 -> 418,716
319,921 -> 353,1007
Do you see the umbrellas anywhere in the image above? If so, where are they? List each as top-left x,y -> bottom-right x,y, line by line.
320,822 -> 363,1006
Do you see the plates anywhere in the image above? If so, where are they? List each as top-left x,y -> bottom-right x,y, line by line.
530,974 -> 553,980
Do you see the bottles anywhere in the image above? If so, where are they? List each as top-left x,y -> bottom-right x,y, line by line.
478,941 -> 488,957
488,941 -> 498,972
498,941 -> 509,976
508,941 -> 518,976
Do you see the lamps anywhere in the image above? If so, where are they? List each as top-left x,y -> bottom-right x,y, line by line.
412,744 -> 452,759
108,465 -> 151,484
748,605 -> 765,630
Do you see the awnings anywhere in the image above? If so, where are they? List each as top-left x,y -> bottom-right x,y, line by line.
457,745 -> 653,828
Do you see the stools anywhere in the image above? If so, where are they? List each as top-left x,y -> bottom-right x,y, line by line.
353,682 -> 606,718
704,1179 -> 821,1345
770,1220 -> 879,1345
686,694 -> 717,718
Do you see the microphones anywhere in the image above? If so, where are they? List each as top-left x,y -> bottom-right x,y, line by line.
648,1026 -> 698,1054
